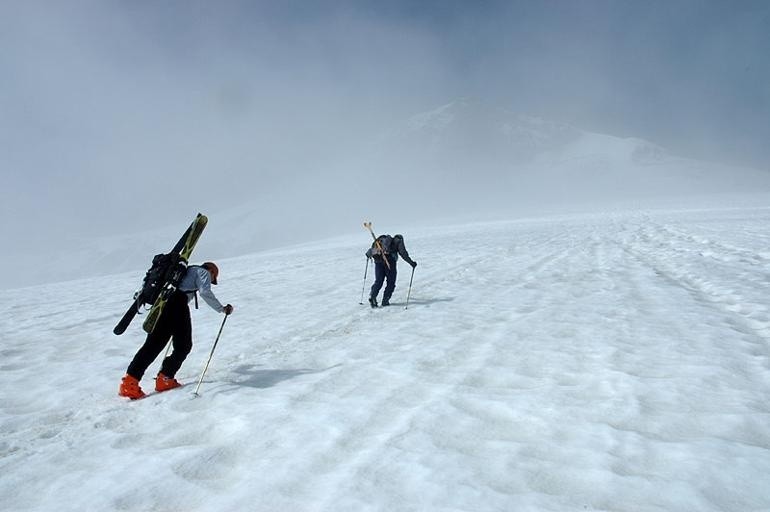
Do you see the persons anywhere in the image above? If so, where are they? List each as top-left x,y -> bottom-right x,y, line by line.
365,234 -> 416,309
118,262 -> 233,399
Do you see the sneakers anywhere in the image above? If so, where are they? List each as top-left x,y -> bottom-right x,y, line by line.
369,296 -> 389,308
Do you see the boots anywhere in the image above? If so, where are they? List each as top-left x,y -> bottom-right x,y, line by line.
119,374 -> 145,399
155,373 -> 180,391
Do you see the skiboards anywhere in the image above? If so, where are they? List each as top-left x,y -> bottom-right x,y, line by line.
113,213 -> 208,335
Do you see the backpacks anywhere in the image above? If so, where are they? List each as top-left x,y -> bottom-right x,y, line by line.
142,253 -> 203,305
371,235 -> 397,261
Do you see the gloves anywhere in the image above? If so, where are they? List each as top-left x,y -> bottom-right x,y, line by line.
223,306 -> 233,314
411,262 -> 417,267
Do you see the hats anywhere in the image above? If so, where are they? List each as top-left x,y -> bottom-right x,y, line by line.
202,263 -> 218,285
396,234 -> 403,241
